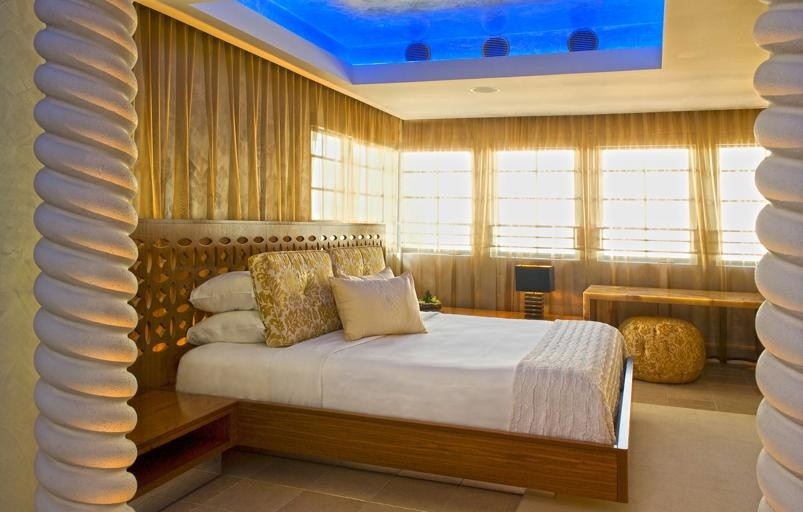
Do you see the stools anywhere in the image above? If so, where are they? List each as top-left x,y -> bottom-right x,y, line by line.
619,315 -> 706,384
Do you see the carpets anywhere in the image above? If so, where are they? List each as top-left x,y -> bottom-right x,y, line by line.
514,401 -> 763,512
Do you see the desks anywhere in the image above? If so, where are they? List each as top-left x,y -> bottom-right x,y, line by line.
583,285 -> 765,364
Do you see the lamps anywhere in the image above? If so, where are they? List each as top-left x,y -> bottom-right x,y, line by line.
515,265 -> 554,319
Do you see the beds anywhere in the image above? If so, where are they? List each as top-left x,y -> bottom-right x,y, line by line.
176,313 -> 634,502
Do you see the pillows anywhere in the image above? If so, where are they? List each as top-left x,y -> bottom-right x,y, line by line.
186,247 -> 428,348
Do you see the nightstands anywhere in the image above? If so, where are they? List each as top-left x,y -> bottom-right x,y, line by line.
127,390 -> 237,512
